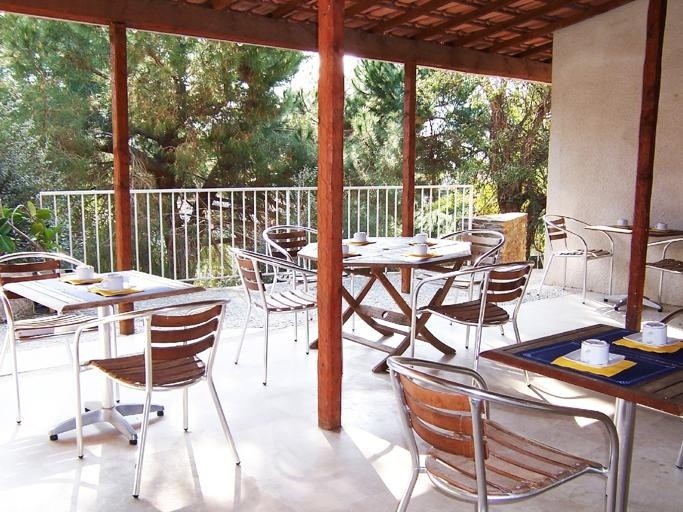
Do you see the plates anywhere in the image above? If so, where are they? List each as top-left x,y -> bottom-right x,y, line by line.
95,283 -> 136,293
624,332 -> 682,347
563,348 -> 626,368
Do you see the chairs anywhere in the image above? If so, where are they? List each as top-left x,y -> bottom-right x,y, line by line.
230,248 -> 320,386
644,239 -> 683,311
416,229 -> 505,348
536,215 -> 614,305
410,261 -> 534,388
660,308 -> 682,469
73,299 -> 240,498
263,226 -> 354,342
0,251 -> 116,423
386,356 -> 619,511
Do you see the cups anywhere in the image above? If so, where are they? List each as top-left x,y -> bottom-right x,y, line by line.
409,242 -> 428,256
617,217 -> 628,225
342,244 -> 350,255
100,274 -> 124,289
76,265 -> 94,279
657,222 -> 668,229
354,232 -> 367,242
413,233 -> 428,244
643,321 -> 667,345
581,339 -> 609,364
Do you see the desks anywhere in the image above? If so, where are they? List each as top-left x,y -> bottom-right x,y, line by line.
297,238 -> 471,372
583,225 -> 683,312
3,270 -> 206,445
479,323 -> 683,511
